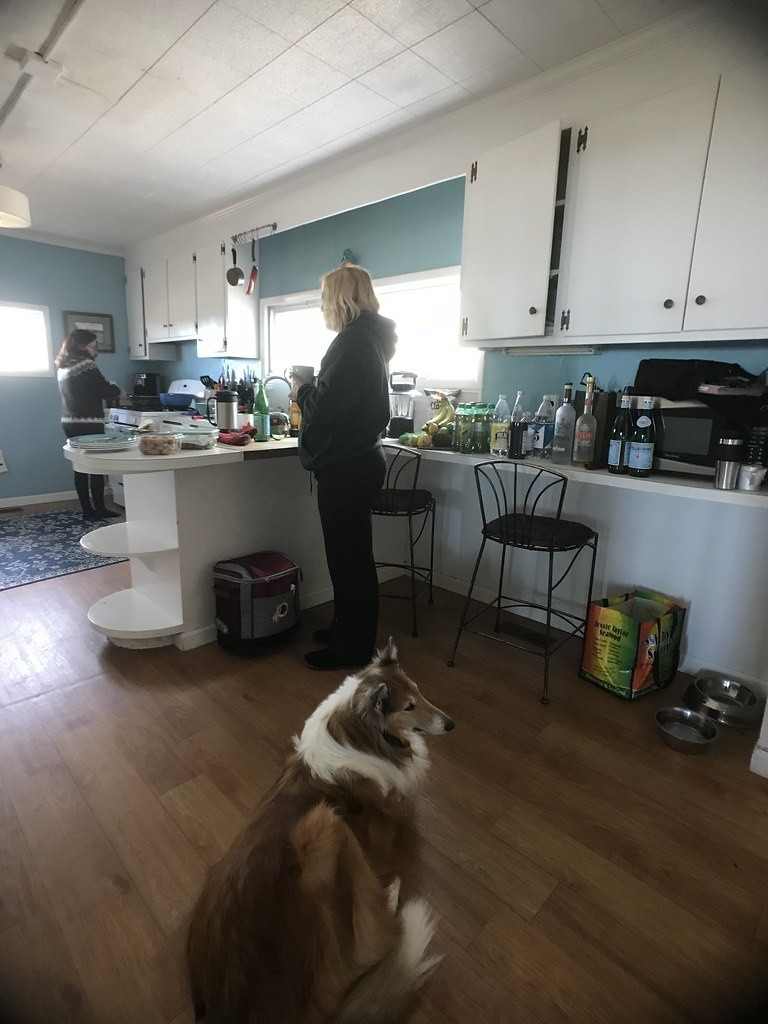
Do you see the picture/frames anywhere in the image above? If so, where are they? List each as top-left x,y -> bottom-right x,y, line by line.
63,310 -> 116,353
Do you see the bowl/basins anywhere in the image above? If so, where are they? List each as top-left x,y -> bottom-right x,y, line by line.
204,388 -> 225,403
655,706 -> 719,751
196,403 -> 216,414
684,672 -> 760,727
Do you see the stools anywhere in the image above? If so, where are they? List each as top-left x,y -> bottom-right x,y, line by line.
447,458 -> 600,706
368,444 -> 435,640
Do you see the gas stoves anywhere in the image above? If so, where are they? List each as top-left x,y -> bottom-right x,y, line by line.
103,379 -> 216,427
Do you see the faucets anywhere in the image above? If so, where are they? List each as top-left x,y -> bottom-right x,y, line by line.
264,375 -> 293,407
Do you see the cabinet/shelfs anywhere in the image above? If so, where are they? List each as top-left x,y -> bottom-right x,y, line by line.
124,267 -> 177,362
459,116 -> 572,347
562,54 -> 768,346
144,241 -> 197,344
195,238 -> 260,359
72,462 -> 183,649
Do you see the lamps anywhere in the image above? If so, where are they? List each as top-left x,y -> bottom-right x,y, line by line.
0,183 -> 32,229
503,344 -> 602,356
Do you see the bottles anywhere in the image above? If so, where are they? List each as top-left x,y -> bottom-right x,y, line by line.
452,391 -> 553,459
253,384 -> 270,442
551,382 -> 576,463
607,395 -> 655,477
570,377 -> 598,468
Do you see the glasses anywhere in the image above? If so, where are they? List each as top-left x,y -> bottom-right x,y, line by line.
87,345 -> 98,351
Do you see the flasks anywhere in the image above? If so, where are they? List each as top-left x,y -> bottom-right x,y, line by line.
208,390 -> 239,433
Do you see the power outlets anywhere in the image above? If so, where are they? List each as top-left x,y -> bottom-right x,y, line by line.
549,394 -> 560,412
0,449 -> 8,474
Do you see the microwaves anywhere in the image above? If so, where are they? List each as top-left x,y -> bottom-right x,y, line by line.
634,376 -> 768,480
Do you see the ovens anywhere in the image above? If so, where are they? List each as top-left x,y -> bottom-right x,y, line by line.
101,422 -> 163,507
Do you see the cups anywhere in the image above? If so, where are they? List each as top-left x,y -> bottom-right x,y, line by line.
714,435 -> 763,491
284,365 -> 314,390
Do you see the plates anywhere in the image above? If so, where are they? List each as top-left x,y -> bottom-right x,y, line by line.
166,428 -> 221,450
67,434 -> 139,451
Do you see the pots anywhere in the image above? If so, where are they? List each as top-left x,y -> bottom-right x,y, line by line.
123,392 -> 162,407
227,248 -> 245,286
246,239 -> 258,296
159,393 -> 193,407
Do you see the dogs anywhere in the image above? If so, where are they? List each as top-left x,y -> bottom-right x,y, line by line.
185,638 -> 458,1024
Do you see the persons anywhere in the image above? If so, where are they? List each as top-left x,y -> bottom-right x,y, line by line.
54,328 -> 127,522
288,263 -> 398,670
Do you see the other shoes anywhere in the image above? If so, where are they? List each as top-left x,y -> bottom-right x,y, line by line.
314,629 -> 335,647
305,649 -> 371,671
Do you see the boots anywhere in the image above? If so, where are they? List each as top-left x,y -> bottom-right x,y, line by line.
91,487 -> 122,518
77,487 -> 106,521
